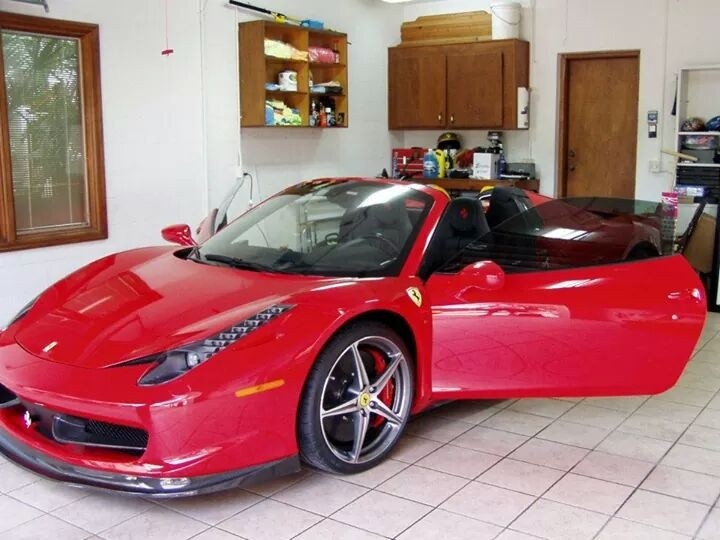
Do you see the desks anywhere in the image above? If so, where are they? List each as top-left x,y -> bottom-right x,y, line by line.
409,176 -> 530,192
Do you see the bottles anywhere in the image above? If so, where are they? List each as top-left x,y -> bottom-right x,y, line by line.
423,148 -> 445,179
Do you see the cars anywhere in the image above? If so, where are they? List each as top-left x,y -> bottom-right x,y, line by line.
0,171 -> 708,502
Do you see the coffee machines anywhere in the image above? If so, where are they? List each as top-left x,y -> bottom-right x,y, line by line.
487,131 -> 504,154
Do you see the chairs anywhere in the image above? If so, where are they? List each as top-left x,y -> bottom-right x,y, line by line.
485,184 -> 545,275
349,188 -> 414,257
422,196 -> 492,285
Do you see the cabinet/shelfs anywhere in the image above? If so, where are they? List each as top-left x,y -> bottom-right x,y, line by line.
387,38 -> 530,131
309,29 -> 350,129
238,19 -> 308,129
674,63 -> 720,238
